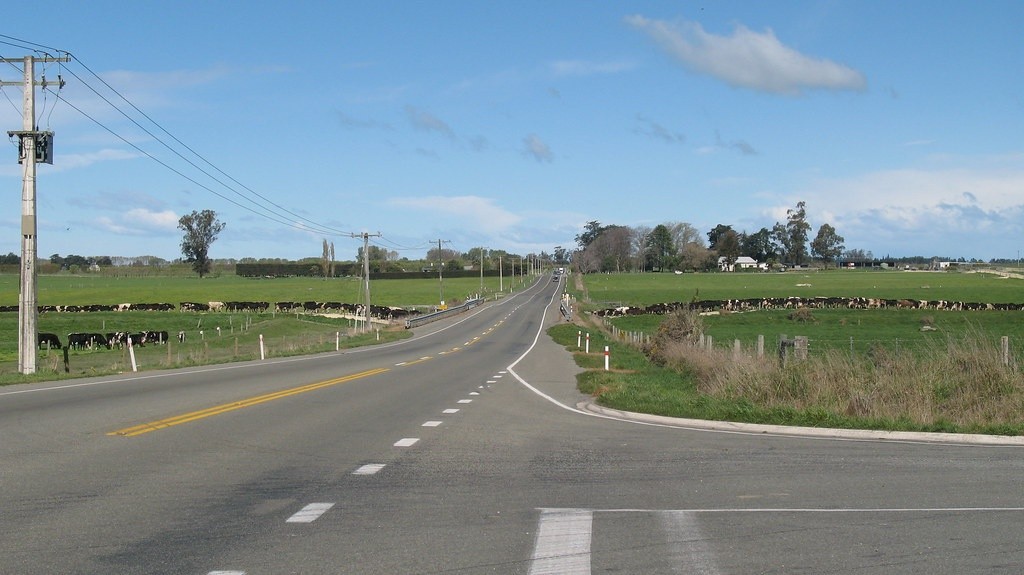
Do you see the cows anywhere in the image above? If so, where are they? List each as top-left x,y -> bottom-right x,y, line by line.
179,301 -> 270,313
274,300 -> 422,322
0,303 -> 176,313
37,330 -> 169,351
583,295 -> 1024,319
176,330 -> 187,343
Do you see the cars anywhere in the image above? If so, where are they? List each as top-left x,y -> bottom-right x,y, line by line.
553,268 -> 565,283
